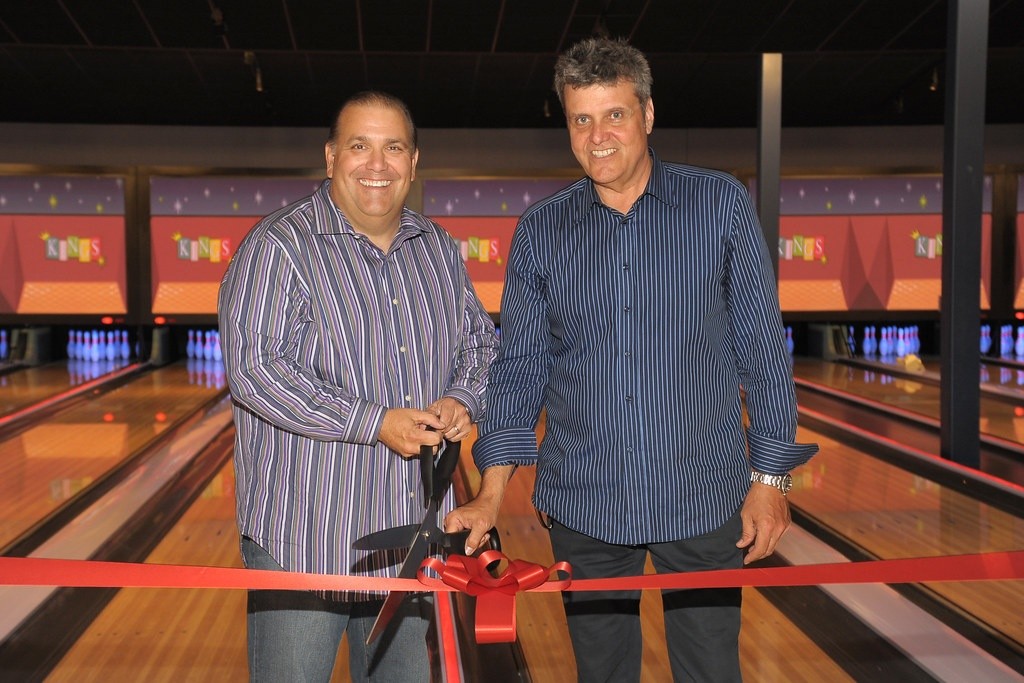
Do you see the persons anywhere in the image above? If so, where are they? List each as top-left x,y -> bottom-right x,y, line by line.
442,39 -> 819,683
218,90 -> 500,683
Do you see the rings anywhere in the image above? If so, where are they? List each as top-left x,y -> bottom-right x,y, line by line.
454,426 -> 460,434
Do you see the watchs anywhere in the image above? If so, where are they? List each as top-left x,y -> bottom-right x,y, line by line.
750,472 -> 793,495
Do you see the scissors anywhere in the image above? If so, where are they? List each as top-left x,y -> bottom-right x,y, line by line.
351,418 -> 502,645
